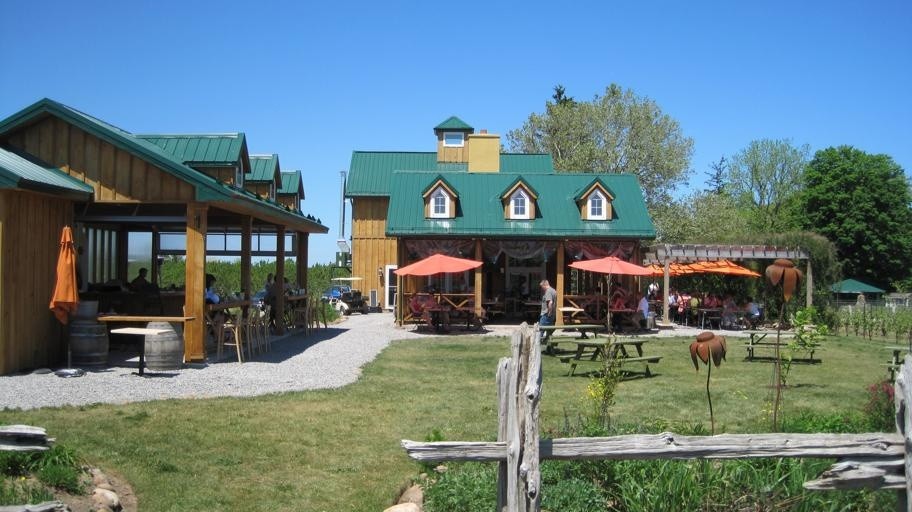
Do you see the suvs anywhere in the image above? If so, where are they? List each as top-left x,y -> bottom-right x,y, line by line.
321,285 -> 351,299
251,290 -> 267,309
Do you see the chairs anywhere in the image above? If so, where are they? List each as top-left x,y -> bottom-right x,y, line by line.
183,292 -> 328,363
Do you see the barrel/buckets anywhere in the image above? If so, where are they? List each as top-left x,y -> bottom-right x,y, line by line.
70,320 -> 111,367
145,322 -> 186,372
78,299 -> 99,318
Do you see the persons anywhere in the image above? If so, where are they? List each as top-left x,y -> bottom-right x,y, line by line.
408,292 -> 435,330
130,267 -> 150,291
538,279 -> 558,345
265,273 -> 294,336
205,273 -> 232,343
574,279 -> 760,333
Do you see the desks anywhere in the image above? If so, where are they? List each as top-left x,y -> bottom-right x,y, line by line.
482,300 -> 542,324
96,314 -> 196,378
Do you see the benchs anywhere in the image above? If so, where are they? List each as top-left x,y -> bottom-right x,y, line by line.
647,295 -> 764,330
413,305 -> 488,333
742,331 -> 822,366
879,345 -> 912,382
558,306 -> 650,333
535,323 -> 664,378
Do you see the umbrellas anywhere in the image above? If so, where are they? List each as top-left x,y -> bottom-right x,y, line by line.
642,257 -> 761,330
829,278 -> 887,295
566,254 -> 654,333
393,250 -> 483,330
49,222 -> 86,369
